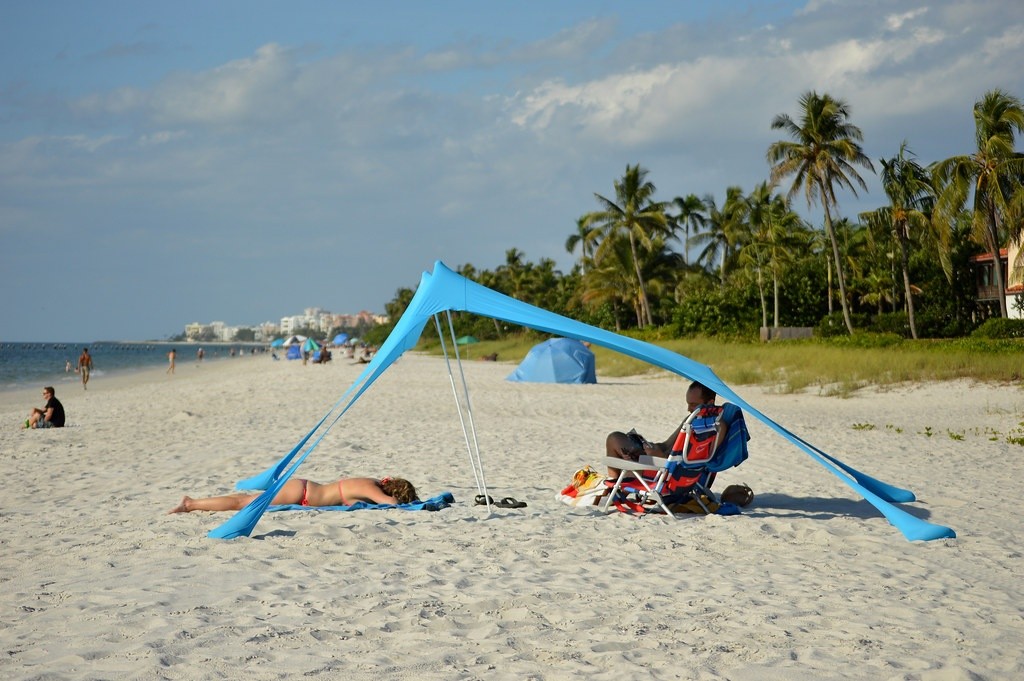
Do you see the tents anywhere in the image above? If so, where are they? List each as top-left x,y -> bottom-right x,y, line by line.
287,345 -> 302,359
206,261 -> 955,543
504,338 -> 597,384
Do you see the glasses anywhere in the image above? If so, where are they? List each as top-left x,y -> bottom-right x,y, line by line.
43,392 -> 48,396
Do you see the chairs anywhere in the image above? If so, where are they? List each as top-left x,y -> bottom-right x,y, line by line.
602,403 -> 750,518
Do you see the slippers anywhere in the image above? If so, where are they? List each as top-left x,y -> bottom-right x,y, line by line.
471,493 -> 494,507
493,496 -> 528,508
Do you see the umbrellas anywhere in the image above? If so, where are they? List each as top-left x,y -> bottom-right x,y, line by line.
271,333 -> 359,351
453,335 -> 480,361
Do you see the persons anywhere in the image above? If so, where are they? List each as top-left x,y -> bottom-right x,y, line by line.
197,346 -> 278,362
77,348 -> 93,390
606,380 -> 718,479
65,361 -> 79,373
581,340 -> 591,347
21,386 -> 65,429
166,348 -> 176,374
316,340 -> 377,364
169,477 -> 417,513
